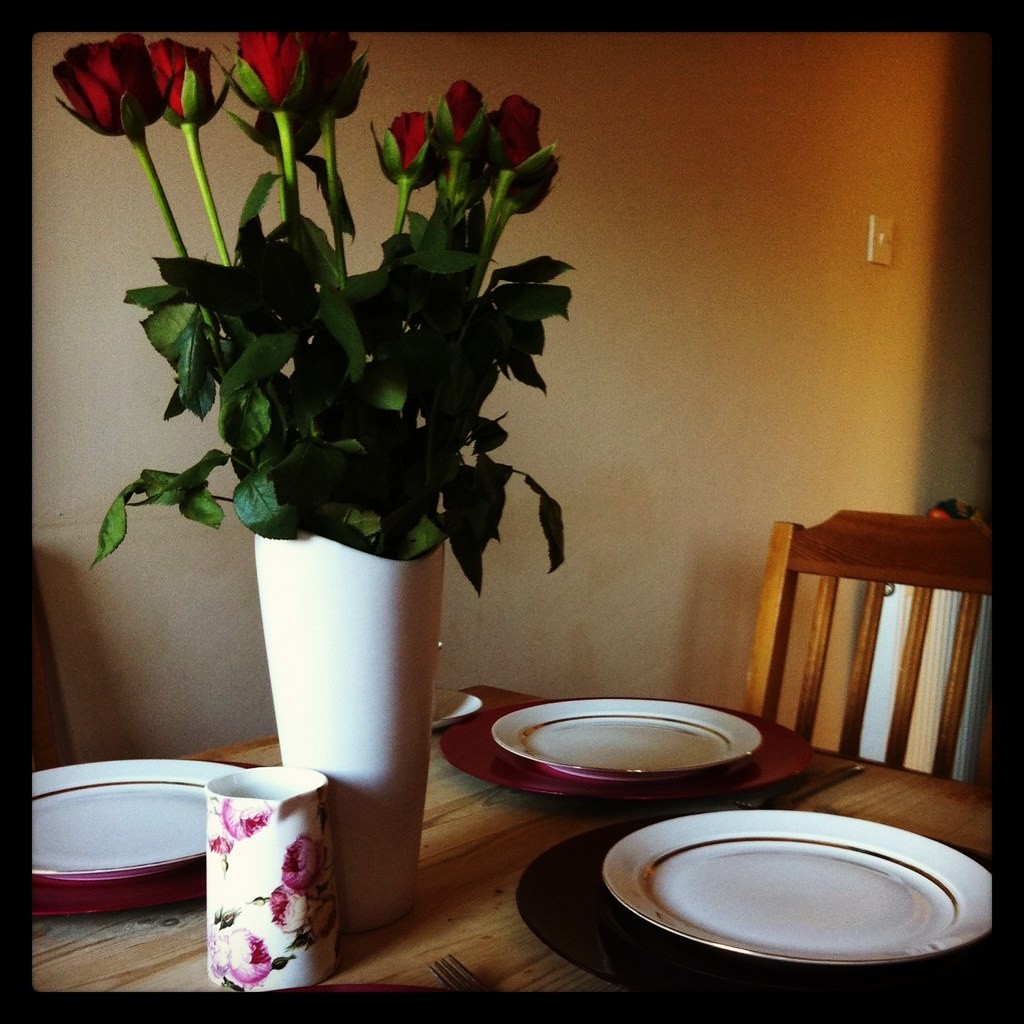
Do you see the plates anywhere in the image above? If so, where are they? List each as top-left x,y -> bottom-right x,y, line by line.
31,757 -> 289,919
515,821 -> 1003,990
428,687 -> 484,734
32,757 -> 263,881
438,693 -> 815,799
602,809 -> 993,965
491,698 -> 764,782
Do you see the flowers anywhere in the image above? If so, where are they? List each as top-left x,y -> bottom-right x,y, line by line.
37,34 -> 573,594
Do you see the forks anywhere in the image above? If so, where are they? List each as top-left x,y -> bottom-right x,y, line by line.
429,952 -> 495,994
733,765 -> 868,810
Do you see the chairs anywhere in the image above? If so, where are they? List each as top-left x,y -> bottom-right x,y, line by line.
744,507 -> 992,787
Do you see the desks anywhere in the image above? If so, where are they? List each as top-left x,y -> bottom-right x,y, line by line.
31,682 -> 993,988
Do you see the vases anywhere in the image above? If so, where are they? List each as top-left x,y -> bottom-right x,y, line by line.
252,522 -> 444,929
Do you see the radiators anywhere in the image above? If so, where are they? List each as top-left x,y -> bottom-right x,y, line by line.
862,575 -> 983,780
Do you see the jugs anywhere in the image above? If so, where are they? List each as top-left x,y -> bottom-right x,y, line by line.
202,766 -> 340,992
